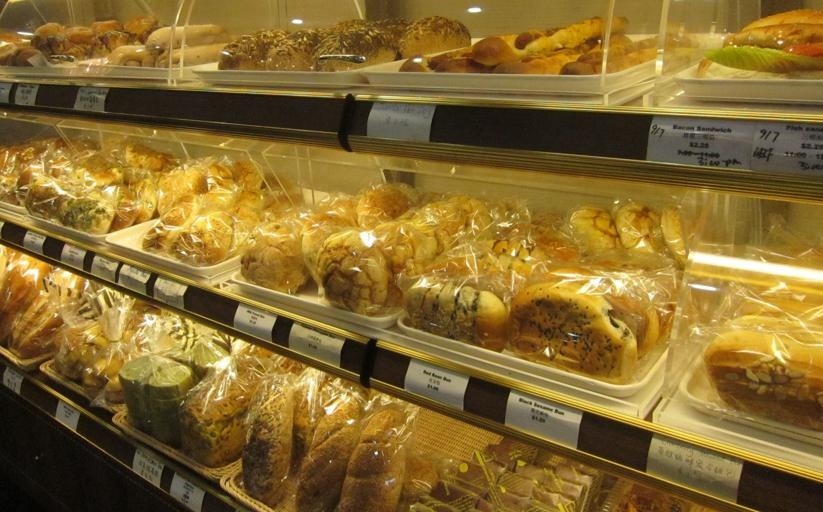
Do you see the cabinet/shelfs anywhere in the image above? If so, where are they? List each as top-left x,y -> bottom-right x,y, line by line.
0,68 -> 823,512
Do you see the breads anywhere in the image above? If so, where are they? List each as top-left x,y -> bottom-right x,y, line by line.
694,9 -> 823,79
1,247 -> 98,360
239,184 -> 514,316
219,16 -> 471,71
1,14 -> 162,69
1,138 -> 97,206
118,339 -> 231,447
402,202 -> 691,383
705,242 -> 822,433
408,437 -> 601,512
141,155 -> 304,266
26,141 -> 179,235
53,319 -> 179,403
399,14 -> 693,75
179,342 -> 308,466
239,366 -> 419,512
106,23 -> 231,68
